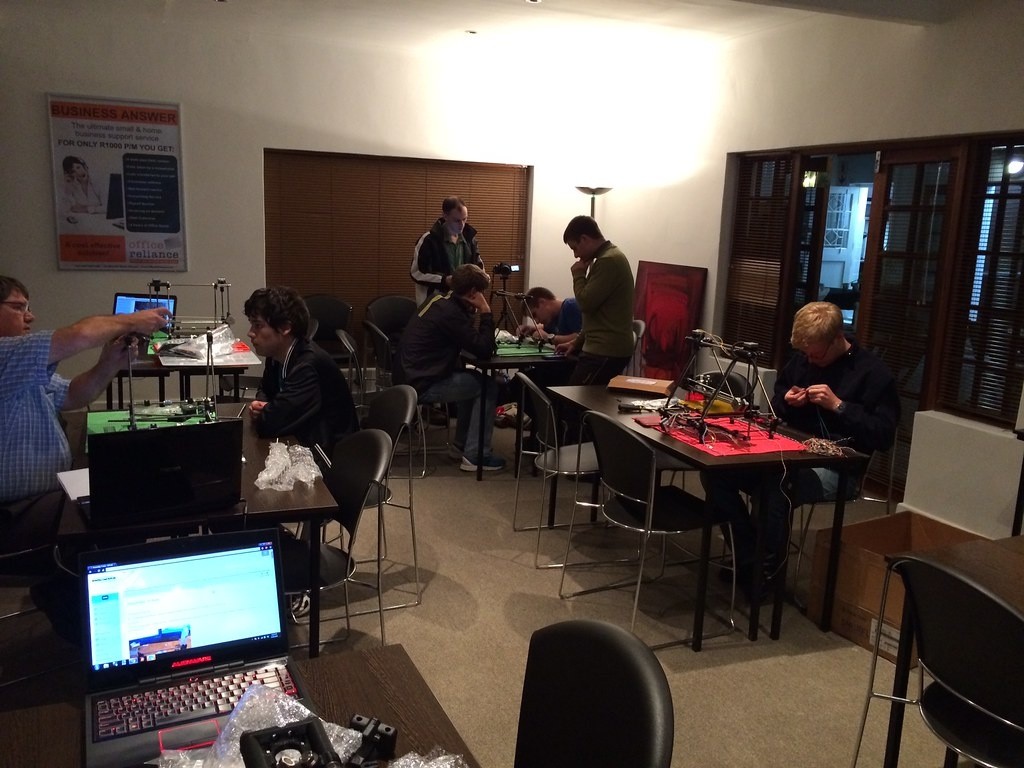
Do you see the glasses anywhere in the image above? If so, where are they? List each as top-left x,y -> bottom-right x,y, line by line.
797,341 -> 832,361
1,300 -> 31,312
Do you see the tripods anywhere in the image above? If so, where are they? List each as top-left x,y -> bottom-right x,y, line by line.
495,276 -> 517,334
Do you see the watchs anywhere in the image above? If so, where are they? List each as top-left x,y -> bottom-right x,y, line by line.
548,333 -> 555,345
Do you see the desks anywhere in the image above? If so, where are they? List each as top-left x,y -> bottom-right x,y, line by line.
546,382 -> 869,652
105,331 -> 250,409
56,402 -> 339,659
0,644 -> 481,768
468,353 -> 565,481
884,535 -> 1024,768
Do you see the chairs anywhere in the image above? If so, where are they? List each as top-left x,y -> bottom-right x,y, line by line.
0,293 -> 1024,768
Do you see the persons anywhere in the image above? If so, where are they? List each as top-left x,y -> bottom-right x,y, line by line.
244,284 -> 358,518
0,276 -> 173,645
62,156 -> 109,214
410,194 -> 486,425
392,265 -> 506,471
515,286 -> 583,445
559,215 -> 634,481
698,300 -> 902,605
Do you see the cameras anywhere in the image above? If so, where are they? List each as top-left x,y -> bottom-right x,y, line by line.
493,262 -> 520,275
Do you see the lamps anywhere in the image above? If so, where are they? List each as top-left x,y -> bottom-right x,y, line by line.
577,187 -> 612,219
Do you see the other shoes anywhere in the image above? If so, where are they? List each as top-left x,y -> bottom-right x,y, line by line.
421,405 -> 449,427
449,444 -> 506,471
718,555 -> 784,600
518,436 -> 534,452
30,574 -> 83,648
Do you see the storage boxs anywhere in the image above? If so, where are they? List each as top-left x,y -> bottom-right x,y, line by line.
806,511 -> 992,669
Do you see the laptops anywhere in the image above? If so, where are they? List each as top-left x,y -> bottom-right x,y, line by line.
113,293 -> 177,334
76,417 -> 247,524
78,526 -> 319,768
